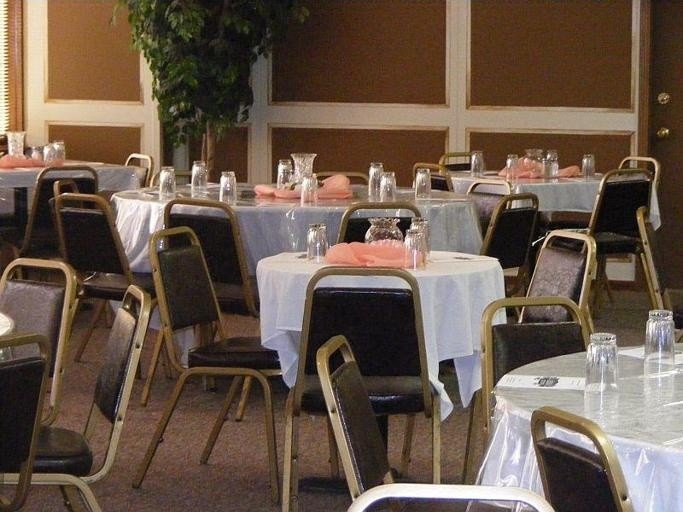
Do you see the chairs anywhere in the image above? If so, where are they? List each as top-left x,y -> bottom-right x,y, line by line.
482,296 -> 593,441
461,228 -> 596,482
316,335 -> 554,510
2,285 -> 150,511
1,258 -> 77,426
133,227 -> 284,503
531,406 -> 633,510
2,152 -> 676,419
2,332 -> 51,510
281,266 -> 441,510
348,484 -> 552,510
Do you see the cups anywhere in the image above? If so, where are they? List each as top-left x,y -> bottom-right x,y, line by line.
218,170 -> 237,203
471,151 -> 484,177
157,166 -> 176,200
645,308 -> 675,366
291,152 -> 316,185
30,140 -> 68,164
581,154 -> 597,179
277,158 -> 292,189
191,160 -> 207,193
306,222 -> 326,262
585,333 -> 619,390
413,169 -> 432,202
368,162 -> 395,201
524,147 -> 558,178
403,217 -> 432,270
6,130 -> 25,161
505,154 -> 518,179
300,174 -> 319,206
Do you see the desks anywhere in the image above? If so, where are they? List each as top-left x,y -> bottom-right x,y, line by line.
466,343 -> 681,510
256,242 -> 506,484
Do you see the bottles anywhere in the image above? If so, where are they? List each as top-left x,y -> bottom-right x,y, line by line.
364,217 -> 405,268
